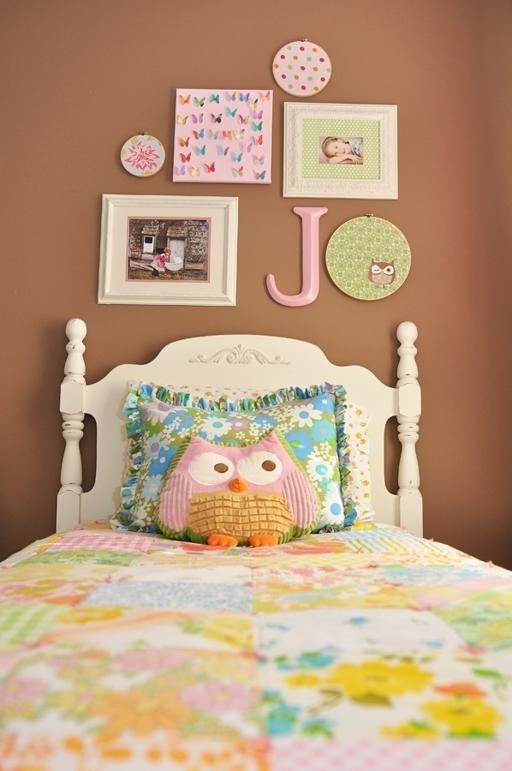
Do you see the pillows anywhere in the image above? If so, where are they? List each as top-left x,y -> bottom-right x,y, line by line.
157,382 -> 372,519
108,377 -> 380,536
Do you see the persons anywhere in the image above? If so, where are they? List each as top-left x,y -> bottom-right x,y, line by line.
321,136 -> 363,164
147,248 -> 171,279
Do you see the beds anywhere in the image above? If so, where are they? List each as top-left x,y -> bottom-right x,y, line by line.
2,316 -> 510,771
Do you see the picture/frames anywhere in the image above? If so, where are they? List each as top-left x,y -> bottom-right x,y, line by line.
94,193 -> 241,308
280,100 -> 400,202
